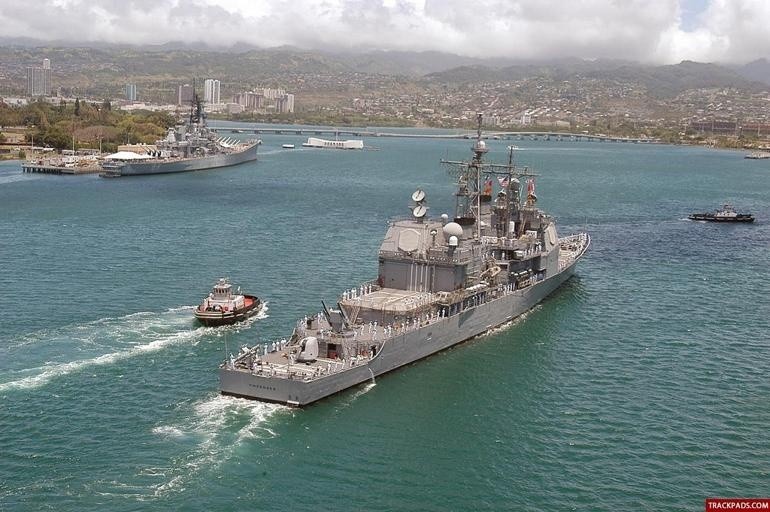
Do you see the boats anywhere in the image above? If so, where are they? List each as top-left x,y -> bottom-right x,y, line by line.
688,203 -> 754,224
303,137 -> 364,151
218,106 -> 591,410
99,79 -> 260,176
282,144 -> 295,149
743,150 -> 770,159
196,279 -> 263,326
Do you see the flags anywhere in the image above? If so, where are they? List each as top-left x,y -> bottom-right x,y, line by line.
500,175 -> 509,187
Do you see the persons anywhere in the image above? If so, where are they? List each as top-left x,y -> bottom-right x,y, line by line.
448,233 -> 588,317
226,277 -> 448,381
